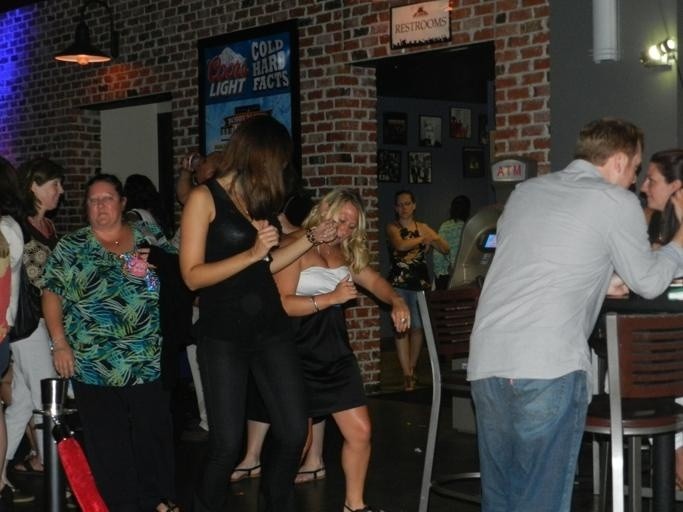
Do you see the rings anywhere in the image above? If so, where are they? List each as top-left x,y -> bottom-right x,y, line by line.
400,318 -> 407,324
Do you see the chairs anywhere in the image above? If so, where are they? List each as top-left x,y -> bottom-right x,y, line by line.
414,279 -> 484,512
581,311 -> 683,512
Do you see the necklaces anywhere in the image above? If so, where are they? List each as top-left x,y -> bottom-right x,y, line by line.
112,239 -> 122,248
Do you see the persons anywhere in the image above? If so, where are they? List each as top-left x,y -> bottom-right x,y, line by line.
168,148 -> 227,440
122,172 -> 173,241
464,116 -> 683,512
228,186 -> 328,486
432,193 -> 474,292
385,188 -> 451,392
174,110 -> 297,512
0,154 -> 27,506
0,154 -> 80,512
639,148 -> 683,252
38,169 -> 185,512
259,182 -> 414,512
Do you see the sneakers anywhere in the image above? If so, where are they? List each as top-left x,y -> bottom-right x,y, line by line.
0,485 -> 36,504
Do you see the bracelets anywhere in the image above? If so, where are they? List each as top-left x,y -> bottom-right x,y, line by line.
309,296 -> 321,313
307,227 -> 322,246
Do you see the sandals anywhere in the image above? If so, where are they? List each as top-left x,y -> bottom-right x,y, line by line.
293,465 -> 327,485
229,462 -> 266,483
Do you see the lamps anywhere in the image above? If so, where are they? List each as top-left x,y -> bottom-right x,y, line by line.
50,20 -> 116,62
639,36 -> 677,72
